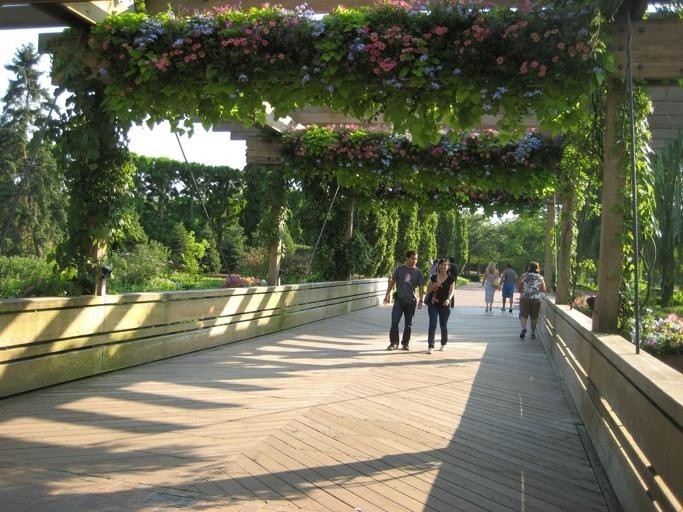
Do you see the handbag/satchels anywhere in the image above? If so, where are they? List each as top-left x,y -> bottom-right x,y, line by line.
423,293 -> 432,305
492,278 -> 500,289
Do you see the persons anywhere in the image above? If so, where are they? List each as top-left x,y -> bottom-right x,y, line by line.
429,259 -> 439,277
481,262 -> 499,312
518,261 -> 546,338
500,263 -> 518,312
383,251 -> 425,350
254,276 -> 260,285
426,258 -> 455,354
448,256 -> 459,308
257,276 -> 265,285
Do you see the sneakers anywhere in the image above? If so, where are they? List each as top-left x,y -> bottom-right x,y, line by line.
427,347 -> 434,354
440,344 -> 446,351
519,329 -> 527,338
485,308 -> 512,313
387,342 -> 398,351
403,343 -> 409,350
530,333 -> 536,339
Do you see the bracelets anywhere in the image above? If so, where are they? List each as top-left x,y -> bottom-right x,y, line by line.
447,298 -> 451,301
419,300 -> 423,303
